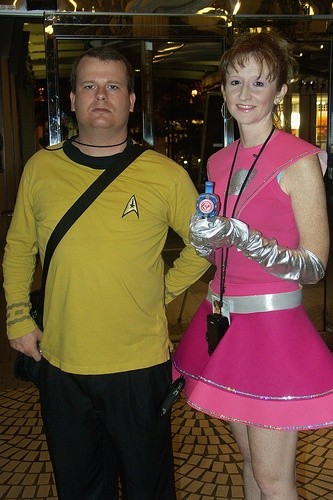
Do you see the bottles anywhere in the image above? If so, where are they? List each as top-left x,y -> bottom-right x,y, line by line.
196,181 -> 220,220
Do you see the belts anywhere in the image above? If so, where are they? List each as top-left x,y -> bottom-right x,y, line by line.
207,279 -> 302,312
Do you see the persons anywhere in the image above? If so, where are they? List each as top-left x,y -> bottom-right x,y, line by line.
2,45 -> 212,500
171,29 -> 333,500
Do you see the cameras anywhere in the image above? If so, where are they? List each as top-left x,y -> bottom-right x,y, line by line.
161,375 -> 185,416
205,313 -> 229,357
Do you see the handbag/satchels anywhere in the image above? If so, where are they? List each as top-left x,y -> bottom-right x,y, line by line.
14,289 -> 42,380
